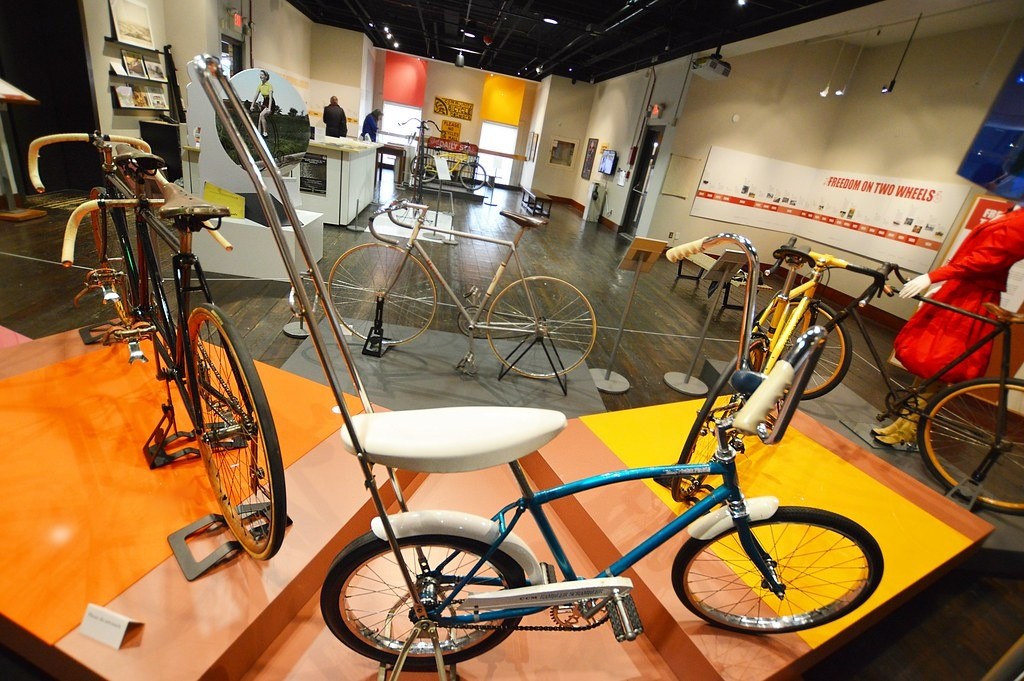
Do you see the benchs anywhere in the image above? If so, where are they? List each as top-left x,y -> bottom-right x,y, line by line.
522,186 -> 553,219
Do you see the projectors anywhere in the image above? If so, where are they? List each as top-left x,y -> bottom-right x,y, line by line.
690,56 -> 732,81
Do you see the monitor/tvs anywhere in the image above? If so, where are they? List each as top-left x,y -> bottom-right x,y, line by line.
598,150 -> 617,175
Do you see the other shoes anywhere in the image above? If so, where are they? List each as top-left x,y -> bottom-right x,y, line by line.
262,132 -> 268,139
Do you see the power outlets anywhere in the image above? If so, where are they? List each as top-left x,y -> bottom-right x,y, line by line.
668,232 -> 673,238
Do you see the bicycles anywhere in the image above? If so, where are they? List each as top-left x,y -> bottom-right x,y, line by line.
396,117 -> 443,204
410,144 -> 487,191
748,260 -> 1024,515
29,134 -> 286,561
671,237 -> 886,501
193,53 -> 885,681
326,198 -> 596,381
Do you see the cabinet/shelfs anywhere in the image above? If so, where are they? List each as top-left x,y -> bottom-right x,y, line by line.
104,36 -> 172,112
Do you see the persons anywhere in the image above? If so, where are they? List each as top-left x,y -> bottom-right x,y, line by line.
156,66 -> 164,78
322,95 -> 348,137
134,57 -> 144,73
584,140 -> 595,171
361,108 -> 383,142
250,69 -> 277,136
870,205 -> 1024,455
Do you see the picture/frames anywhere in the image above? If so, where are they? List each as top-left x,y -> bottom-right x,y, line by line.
581,138 -> 599,180
109,0 -> 156,51
545,135 -> 580,170
120,48 -> 149,80
142,55 -> 168,83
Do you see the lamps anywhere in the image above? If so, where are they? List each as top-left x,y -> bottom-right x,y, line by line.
227,7 -> 248,34
835,29 -> 871,95
455,51 -> 465,67
464,21 -> 476,38
536,65 -> 544,75
971,15 -> 1016,89
820,36 -> 847,97
881,13 -> 923,93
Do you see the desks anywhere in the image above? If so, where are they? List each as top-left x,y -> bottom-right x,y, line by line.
671,252 -> 774,321
374,144 -> 406,186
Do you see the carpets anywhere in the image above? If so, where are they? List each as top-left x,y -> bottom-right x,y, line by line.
363,203 -> 454,243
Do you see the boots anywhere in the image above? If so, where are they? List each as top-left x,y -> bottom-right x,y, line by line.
871,398 -> 917,436
874,393 -> 938,447
